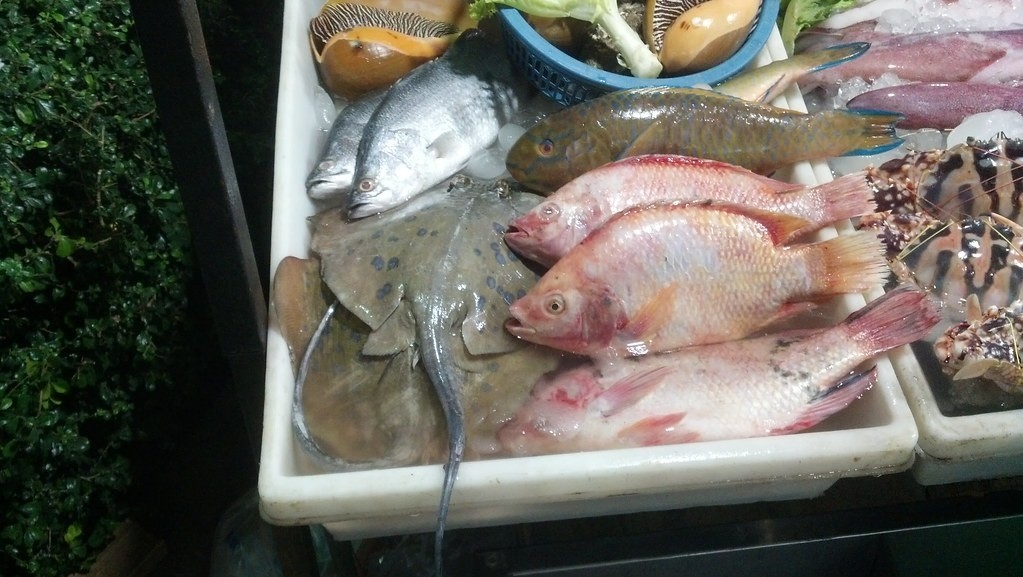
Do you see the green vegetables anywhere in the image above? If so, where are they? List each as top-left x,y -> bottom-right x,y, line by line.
467,0 -> 857,80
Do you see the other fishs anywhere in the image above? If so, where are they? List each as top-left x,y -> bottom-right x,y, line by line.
273,28 -> 942,572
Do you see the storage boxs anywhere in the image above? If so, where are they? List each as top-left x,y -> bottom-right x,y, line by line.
258,0 -> 1023,542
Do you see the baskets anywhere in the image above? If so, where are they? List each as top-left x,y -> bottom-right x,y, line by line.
494,0 -> 781,107
